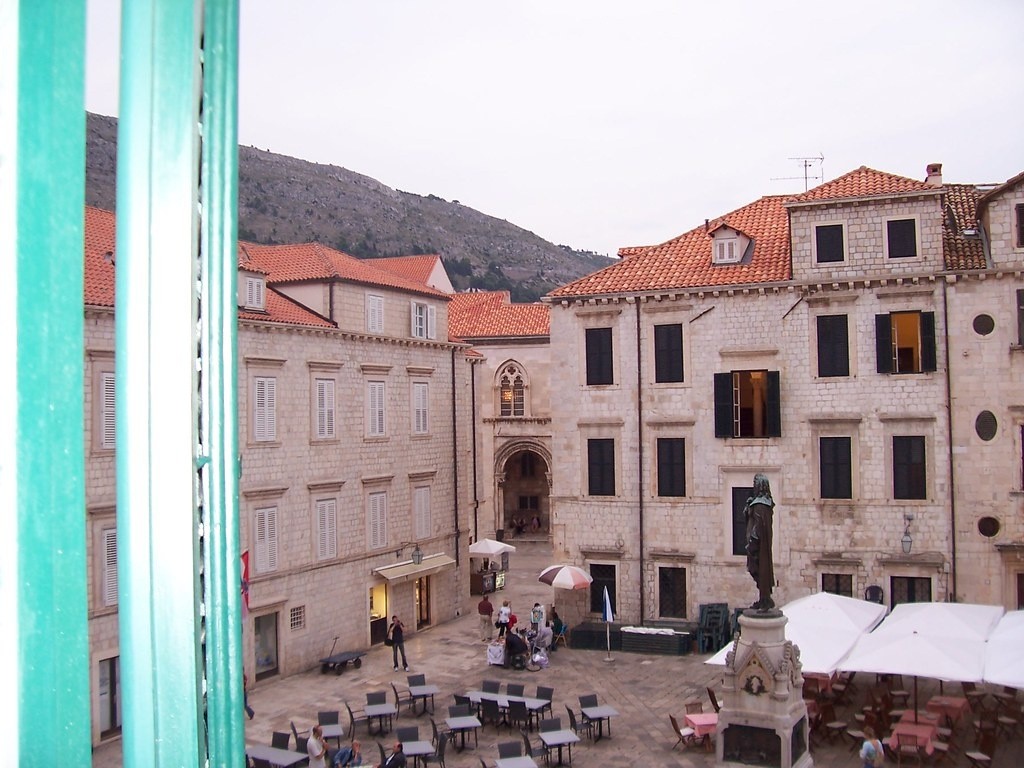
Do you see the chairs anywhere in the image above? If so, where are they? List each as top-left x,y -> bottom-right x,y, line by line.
532,633 -> 553,656
866,585 -> 884,605
809,671 -> 1024,768
510,645 -> 532,670
241,673 -> 603,768
697,603 -> 746,654
669,687 -> 725,752
554,623 -> 569,646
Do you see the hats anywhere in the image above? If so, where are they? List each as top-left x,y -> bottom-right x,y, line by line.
503,600 -> 510,605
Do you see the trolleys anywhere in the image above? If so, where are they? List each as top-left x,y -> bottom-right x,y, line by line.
319,636 -> 368,675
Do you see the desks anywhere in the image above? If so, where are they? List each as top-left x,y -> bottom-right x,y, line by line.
803,673 -> 834,697
580,705 -> 619,743
488,631 -> 531,667
409,685 -> 440,717
462,690 -> 550,732
444,716 -> 483,753
685,713 -> 719,753
308,724 -> 346,756
245,745 -> 310,768
802,698 -> 815,713
537,729 -> 582,768
898,708 -> 945,727
363,703 -> 398,739
401,741 -> 435,768
926,694 -> 970,736
494,755 -> 539,768
889,722 -> 937,757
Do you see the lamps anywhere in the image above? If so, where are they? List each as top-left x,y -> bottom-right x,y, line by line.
394,541 -> 425,564
901,514 -> 914,554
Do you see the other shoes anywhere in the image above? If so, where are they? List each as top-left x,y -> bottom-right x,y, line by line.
404,666 -> 410,672
394,667 -> 397,671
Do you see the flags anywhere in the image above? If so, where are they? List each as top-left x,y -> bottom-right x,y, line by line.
240,552 -> 250,622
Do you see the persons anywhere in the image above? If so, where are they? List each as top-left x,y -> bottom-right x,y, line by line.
530,603 -> 541,633
389,616 -> 409,671
550,612 -> 563,635
243,667 -> 254,720
477,595 -> 494,641
504,631 -> 527,668
510,514 -> 528,534
534,621 -> 553,648
373,741 -> 406,768
530,514 -> 540,533
306,725 -> 329,768
743,473 -> 776,614
859,727 -> 884,768
497,601 -> 511,639
333,739 -> 362,768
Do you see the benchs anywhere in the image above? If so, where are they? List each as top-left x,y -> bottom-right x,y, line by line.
504,523 -> 516,540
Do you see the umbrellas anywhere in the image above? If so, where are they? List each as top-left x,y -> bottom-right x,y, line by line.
468,538 -> 516,572
709,593 -> 1024,726
537,564 -> 594,624
602,586 -> 614,658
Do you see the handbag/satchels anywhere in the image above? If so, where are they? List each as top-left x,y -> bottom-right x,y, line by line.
495,621 -> 500,628
385,639 -> 393,646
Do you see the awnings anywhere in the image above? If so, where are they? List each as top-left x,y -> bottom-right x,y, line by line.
377,555 -> 456,584
